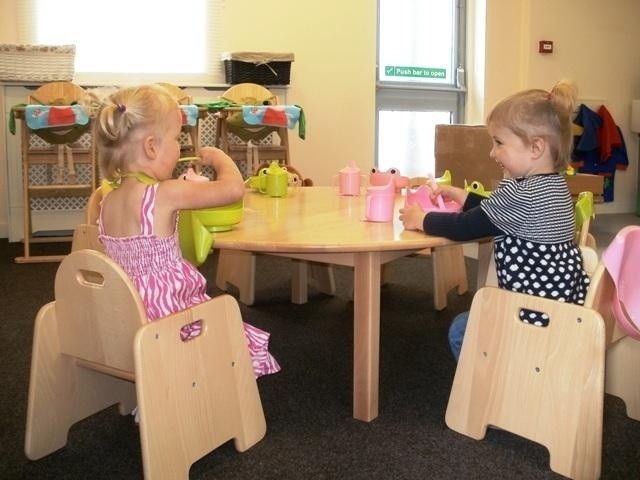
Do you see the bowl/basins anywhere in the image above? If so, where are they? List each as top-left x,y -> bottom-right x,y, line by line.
247,167 -> 288,193
369,168 -> 410,193
405,185 -> 463,213
191,197 -> 246,232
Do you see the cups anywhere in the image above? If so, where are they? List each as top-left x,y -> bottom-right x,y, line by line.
340,161 -> 362,196
365,177 -> 395,223
258,160 -> 288,197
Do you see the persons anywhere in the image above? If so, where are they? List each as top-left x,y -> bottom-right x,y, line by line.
86,86 -> 281,426
398,81 -> 590,367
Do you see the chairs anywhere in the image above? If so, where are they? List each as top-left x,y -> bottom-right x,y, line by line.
480,192 -> 604,294
25,250 -> 268,480
172,105 -> 205,179
216,164 -> 337,307
71,187 -> 104,284
445,225 -> 640,480
13,103 -> 97,264
351,178 -> 469,311
213,105 -> 295,185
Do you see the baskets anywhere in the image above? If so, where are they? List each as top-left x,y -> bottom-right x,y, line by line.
0,43 -> 76,82
224,52 -> 295,86
